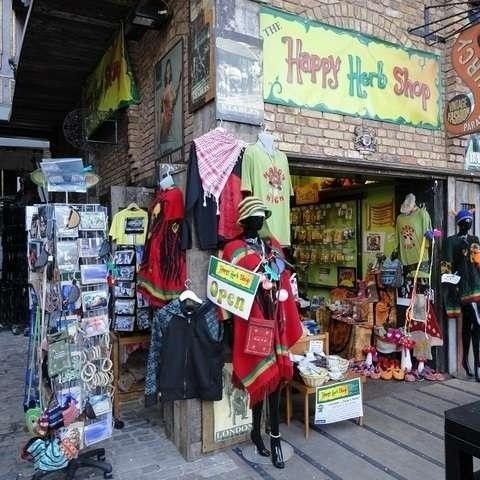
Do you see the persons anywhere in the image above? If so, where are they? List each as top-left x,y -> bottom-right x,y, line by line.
218,196 -> 303,469
440,211 -> 480,382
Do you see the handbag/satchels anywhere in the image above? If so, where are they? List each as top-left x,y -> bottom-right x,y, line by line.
21,396 -> 84,472
244,317 -> 275,357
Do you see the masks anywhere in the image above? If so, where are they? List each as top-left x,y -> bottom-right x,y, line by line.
67,285 -> 80,304
99,240 -> 110,258
85,403 -> 96,419
34,250 -> 48,268
66,206 -> 81,230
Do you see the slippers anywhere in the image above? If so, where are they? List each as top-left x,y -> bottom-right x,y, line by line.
353,362 -> 444,382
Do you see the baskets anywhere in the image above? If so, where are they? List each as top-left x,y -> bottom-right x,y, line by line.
300,368 -> 327,387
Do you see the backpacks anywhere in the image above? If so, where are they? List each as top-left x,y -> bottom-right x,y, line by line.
381,258 -> 403,288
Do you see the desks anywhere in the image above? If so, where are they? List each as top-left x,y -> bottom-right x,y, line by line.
286,379 -> 364,440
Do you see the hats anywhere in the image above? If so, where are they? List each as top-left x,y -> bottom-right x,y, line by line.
456,210 -> 473,223
237,197 -> 272,225
